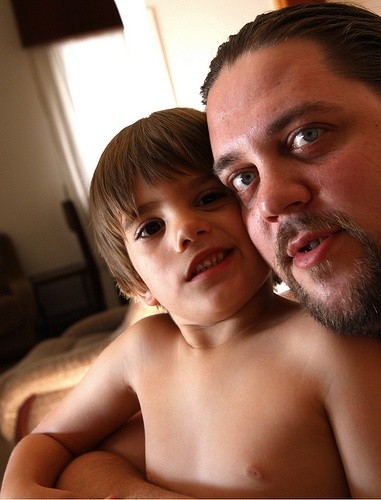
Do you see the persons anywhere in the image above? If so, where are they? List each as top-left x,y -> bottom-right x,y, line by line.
198,3 -> 380,336
0,108 -> 381,500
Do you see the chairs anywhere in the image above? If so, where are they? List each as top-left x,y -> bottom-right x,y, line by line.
30,200 -> 109,343
